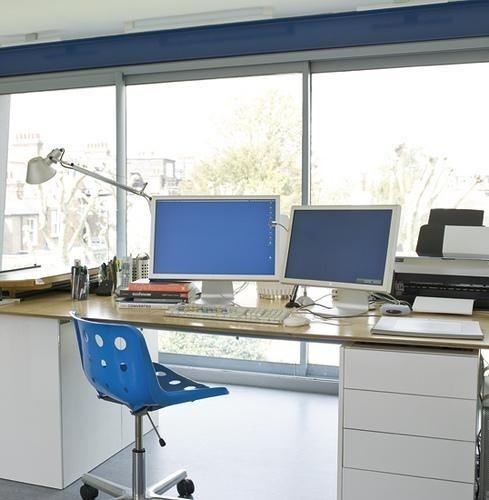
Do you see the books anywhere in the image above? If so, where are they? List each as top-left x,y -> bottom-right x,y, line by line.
369,315 -> 484,340
114,277 -> 198,310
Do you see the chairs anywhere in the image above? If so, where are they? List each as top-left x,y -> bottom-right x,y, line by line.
69,310 -> 228,500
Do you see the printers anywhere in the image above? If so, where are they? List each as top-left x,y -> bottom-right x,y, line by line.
373,209 -> 489,311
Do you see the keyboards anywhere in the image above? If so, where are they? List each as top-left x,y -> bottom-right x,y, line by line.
163,301 -> 290,325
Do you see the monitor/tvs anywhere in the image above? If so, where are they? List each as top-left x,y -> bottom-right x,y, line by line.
280,204 -> 401,318
148,196 -> 280,306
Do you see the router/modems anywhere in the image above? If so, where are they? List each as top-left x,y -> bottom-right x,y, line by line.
381,303 -> 410,315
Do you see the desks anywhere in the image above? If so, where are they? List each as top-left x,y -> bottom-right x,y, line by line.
0,290 -> 489,500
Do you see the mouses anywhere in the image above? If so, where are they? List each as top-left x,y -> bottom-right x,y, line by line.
284,315 -> 311,327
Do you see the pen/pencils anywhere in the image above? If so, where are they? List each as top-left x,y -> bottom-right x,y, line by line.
99,256 -> 121,291
72,259 -> 87,300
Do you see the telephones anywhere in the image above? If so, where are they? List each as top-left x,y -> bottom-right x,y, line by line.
115,254 -> 134,297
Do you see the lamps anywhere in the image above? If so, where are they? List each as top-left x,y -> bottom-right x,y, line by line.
26,147 -> 152,200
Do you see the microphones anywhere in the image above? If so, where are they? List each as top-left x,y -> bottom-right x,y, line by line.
268,220 -> 315,306
